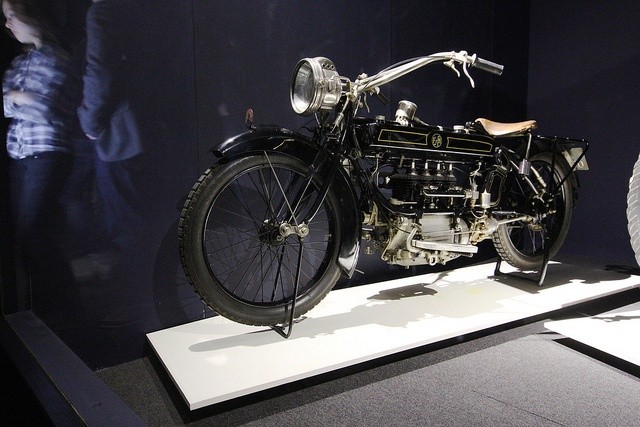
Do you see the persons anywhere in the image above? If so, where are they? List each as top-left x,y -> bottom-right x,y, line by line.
0,1 -> 79,275
77,1 -> 151,273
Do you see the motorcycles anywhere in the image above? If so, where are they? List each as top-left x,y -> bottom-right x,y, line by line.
178,50 -> 589,337
